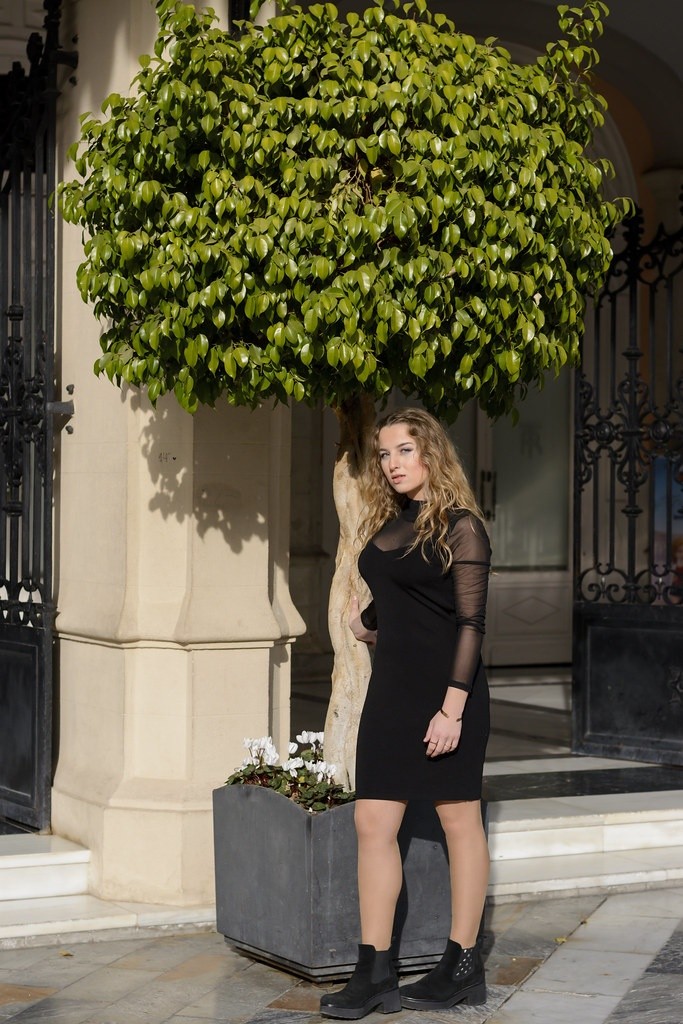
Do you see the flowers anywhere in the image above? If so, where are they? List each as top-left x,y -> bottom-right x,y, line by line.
225,730 -> 356,811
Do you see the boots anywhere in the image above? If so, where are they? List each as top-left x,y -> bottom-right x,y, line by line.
398,939 -> 487,1012
318,940 -> 401,1019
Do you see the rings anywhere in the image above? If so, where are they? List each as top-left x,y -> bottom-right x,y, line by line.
429,741 -> 437,746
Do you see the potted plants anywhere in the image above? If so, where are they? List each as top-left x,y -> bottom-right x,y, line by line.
55,0 -> 640,984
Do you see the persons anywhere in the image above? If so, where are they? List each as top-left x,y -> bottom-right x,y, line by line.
319,407 -> 492,1019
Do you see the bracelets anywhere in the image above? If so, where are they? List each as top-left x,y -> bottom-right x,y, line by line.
440,709 -> 462,722
